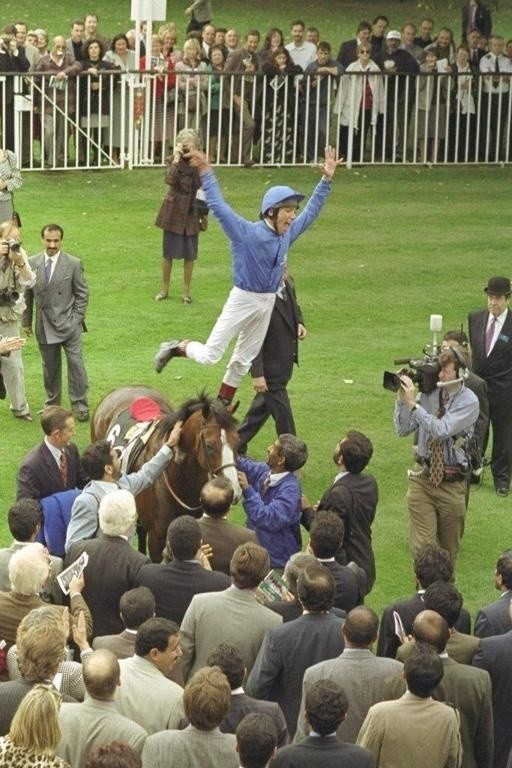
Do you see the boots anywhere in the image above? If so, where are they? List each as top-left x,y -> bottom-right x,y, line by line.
154,339 -> 188,374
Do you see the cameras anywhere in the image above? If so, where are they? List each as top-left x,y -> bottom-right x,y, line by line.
1,240 -> 20,253
182,144 -> 192,160
1,34 -> 15,46
0,287 -> 19,306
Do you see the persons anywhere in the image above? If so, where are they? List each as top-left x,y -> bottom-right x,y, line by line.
185,0 -> 212,33
236,271 -> 307,456
23,224 -> 89,420
1,406 -> 512,768
153,144 -> 343,407
1,150 -> 37,421
395,331 -> 490,585
468,277 -> 512,496
155,129 -> 211,304
1,13 -> 512,168
461,0 -> 492,42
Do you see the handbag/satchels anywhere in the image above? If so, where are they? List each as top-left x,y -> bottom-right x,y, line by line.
181,94 -> 203,114
11,210 -> 22,228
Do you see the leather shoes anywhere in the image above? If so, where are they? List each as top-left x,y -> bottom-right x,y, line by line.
154,293 -> 191,307
76,409 -> 90,423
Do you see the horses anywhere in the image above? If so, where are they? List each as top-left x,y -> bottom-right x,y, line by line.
89,381 -> 244,563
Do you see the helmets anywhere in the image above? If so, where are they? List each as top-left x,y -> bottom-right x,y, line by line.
260,185 -> 305,216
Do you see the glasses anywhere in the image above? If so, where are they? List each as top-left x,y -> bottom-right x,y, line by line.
360,49 -> 370,54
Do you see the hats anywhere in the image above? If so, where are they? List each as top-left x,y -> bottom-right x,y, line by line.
484,277 -> 511,295
387,30 -> 401,41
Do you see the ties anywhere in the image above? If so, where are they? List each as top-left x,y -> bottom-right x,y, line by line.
483,318 -> 495,353
429,397 -> 449,484
492,58 -> 500,88
281,287 -> 288,301
45,259 -> 52,283
59,452 -> 70,486
